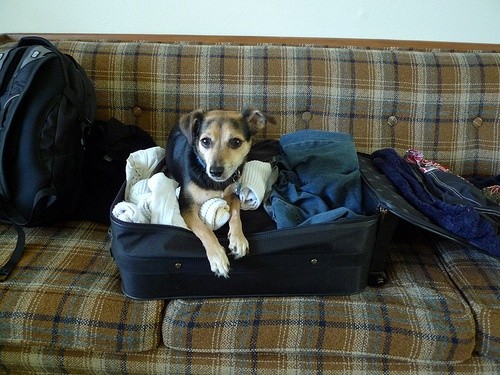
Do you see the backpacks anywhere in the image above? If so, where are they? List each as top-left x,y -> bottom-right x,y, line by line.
0,36 -> 97,282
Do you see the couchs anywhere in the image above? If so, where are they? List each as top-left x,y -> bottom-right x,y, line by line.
0,32 -> 499,375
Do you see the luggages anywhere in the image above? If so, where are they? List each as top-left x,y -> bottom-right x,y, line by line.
107,152 -> 500,299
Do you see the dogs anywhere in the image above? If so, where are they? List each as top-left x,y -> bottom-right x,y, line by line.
164,107 -> 278,281
